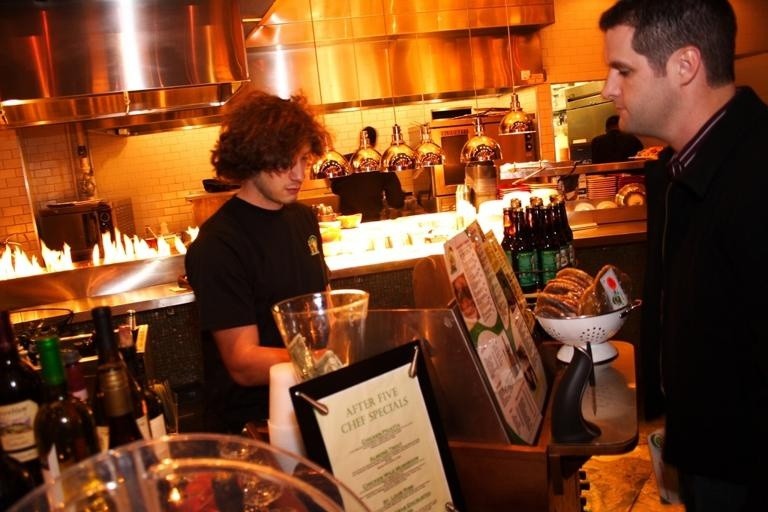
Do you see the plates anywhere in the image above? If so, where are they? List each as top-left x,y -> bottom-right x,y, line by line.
577,172 -> 645,200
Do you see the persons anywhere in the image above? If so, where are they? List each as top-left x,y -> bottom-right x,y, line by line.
589,114 -> 644,176
184,91 -> 332,454
599,0 -> 767,511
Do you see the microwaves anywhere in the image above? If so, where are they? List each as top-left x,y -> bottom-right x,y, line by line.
39,195 -> 136,262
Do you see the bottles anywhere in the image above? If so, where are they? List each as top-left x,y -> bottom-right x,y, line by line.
500,193 -> 579,304
0,300 -> 148,482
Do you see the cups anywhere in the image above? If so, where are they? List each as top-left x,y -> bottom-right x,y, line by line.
271,287 -> 370,383
264,361 -> 308,476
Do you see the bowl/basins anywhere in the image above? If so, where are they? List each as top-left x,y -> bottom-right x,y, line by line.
318,221 -> 343,228
321,226 -> 342,243
5,431 -> 376,512
597,200 -> 617,209
615,182 -> 647,208
338,212 -> 363,228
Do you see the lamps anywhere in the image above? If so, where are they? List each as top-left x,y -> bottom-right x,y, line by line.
309,0 -> 353,181
497,0 -> 537,137
381,0 -> 416,173
459,0 -> 504,164
414,0 -> 448,170
349,0 -> 383,174
